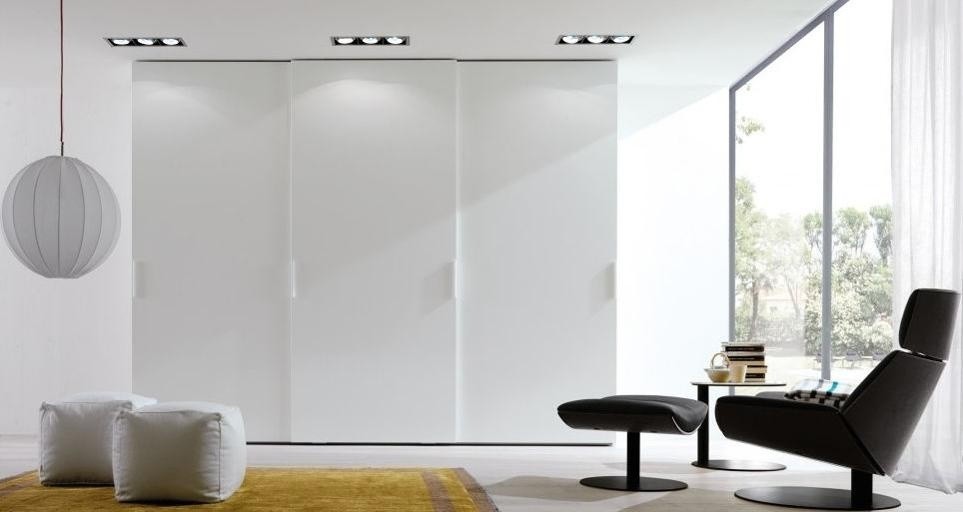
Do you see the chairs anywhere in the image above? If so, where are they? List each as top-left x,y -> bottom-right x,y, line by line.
715,289 -> 961,511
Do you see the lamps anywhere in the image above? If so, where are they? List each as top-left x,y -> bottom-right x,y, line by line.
0,0 -> 121,279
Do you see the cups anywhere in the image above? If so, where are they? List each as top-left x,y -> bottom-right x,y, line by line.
729,363 -> 746,382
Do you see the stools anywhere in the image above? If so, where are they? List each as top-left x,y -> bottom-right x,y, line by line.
112,402 -> 246,503
560,396 -> 708,492
38,399 -> 156,487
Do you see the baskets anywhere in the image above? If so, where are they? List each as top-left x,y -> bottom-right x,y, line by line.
703,349 -> 732,383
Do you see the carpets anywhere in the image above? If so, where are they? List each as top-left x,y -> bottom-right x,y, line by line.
0,466 -> 500,512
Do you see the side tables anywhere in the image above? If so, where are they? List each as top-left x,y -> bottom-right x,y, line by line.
693,381 -> 786,471
